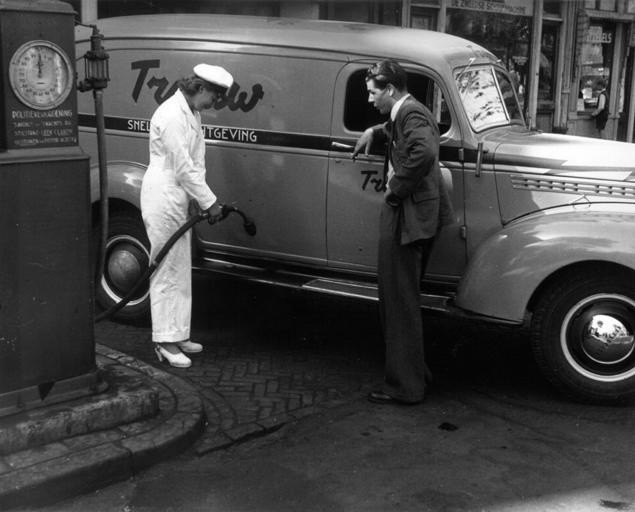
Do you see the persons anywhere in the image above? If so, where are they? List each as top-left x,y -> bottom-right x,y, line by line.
138,64 -> 233,369
350,58 -> 458,404
591,79 -> 610,139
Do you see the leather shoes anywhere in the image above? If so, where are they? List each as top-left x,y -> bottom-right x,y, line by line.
366,381 -> 429,404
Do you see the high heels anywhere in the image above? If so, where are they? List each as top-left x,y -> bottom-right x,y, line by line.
154,340 -> 204,369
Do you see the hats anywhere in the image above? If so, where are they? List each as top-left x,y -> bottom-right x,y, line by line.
193,63 -> 234,89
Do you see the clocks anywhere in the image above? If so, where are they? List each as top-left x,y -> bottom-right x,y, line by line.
9,39 -> 76,111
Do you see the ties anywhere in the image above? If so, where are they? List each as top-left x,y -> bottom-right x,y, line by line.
382,117 -> 395,192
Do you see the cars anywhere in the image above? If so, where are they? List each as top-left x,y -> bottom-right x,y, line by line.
74,13 -> 635,406
